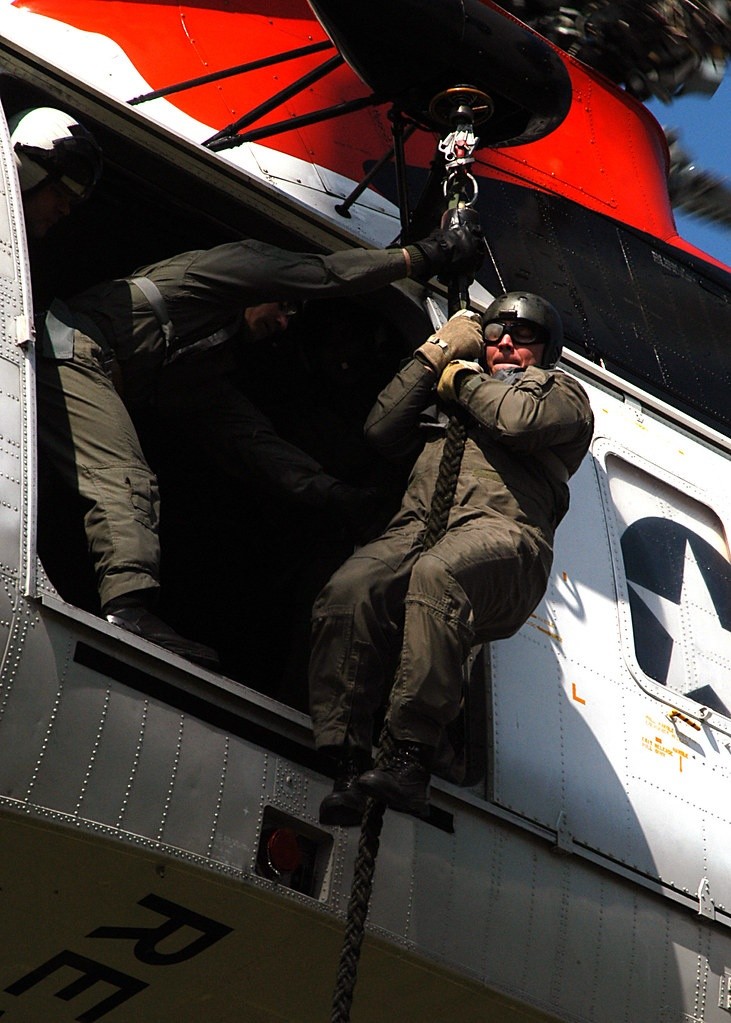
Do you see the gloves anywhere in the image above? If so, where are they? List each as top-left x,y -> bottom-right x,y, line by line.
413,309 -> 485,373
328,481 -> 401,530
434,358 -> 485,402
413,221 -> 485,274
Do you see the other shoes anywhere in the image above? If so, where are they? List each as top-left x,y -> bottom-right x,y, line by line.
100,595 -> 220,663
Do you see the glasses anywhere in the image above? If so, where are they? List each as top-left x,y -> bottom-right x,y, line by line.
274,294 -> 301,318
482,321 -> 544,347
52,139 -> 101,188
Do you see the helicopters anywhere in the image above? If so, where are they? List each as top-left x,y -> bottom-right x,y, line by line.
0,1 -> 731,1023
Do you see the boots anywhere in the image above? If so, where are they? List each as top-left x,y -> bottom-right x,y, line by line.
318,748 -> 373,826
360,741 -> 433,820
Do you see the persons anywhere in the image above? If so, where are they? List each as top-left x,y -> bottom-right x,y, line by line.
36,208 -> 484,665
310,293 -> 594,827
5,107 -> 102,238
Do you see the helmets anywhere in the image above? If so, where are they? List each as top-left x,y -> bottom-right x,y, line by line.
479,291 -> 564,369
7,106 -> 102,205
